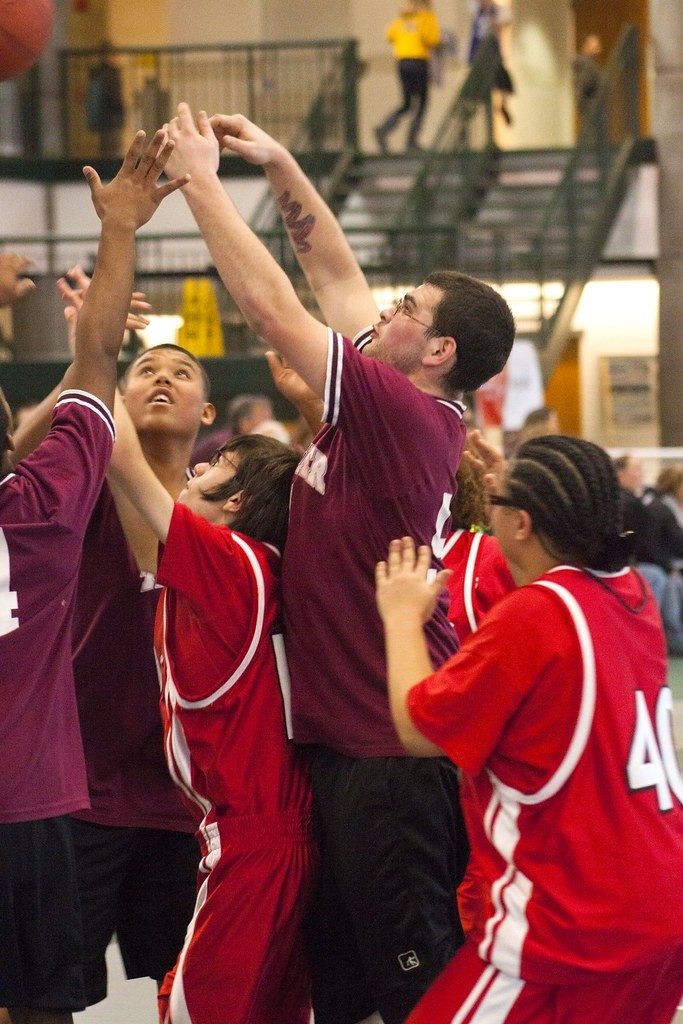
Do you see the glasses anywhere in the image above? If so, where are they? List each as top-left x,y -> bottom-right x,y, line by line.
392,297 -> 442,337
208,449 -> 240,474
482,485 -> 540,536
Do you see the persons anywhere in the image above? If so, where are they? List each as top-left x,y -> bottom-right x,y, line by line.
465,0 -> 513,149
375,435 -> 683,1024
374,0 -> 443,154
0,128 -> 191,1024
0,248 -> 312,1024
56,263 -> 313,1024
615,454 -> 683,656
571,33 -> 610,149
263,345 -> 517,650
87,40 -> 125,159
148,103 -> 515,1024
